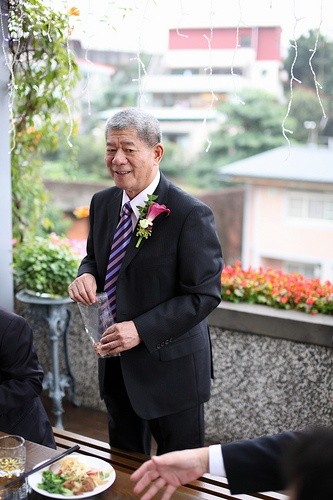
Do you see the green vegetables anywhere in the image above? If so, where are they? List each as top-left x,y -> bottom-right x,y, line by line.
38,469 -> 73,495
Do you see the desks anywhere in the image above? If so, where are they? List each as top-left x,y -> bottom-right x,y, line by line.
0,431 -> 208,500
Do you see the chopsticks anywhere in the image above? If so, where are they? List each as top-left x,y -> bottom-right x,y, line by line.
4,445 -> 80,488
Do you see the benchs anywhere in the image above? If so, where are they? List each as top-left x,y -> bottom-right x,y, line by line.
52,425 -> 288,500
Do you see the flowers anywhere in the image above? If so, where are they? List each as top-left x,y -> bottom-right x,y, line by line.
136,194 -> 170,249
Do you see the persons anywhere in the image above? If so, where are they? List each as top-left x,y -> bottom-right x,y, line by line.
0,309 -> 56,450
130,427 -> 333,500
68,108 -> 223,456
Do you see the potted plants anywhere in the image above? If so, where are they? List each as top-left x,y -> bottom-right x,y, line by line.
12,236 -> 81,323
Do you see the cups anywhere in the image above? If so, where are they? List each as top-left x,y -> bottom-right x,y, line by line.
0,435 -> 27,500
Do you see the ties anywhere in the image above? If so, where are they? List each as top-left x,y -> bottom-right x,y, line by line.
99,200 -> 134,335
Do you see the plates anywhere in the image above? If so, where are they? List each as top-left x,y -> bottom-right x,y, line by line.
27,454 -> 116,500
